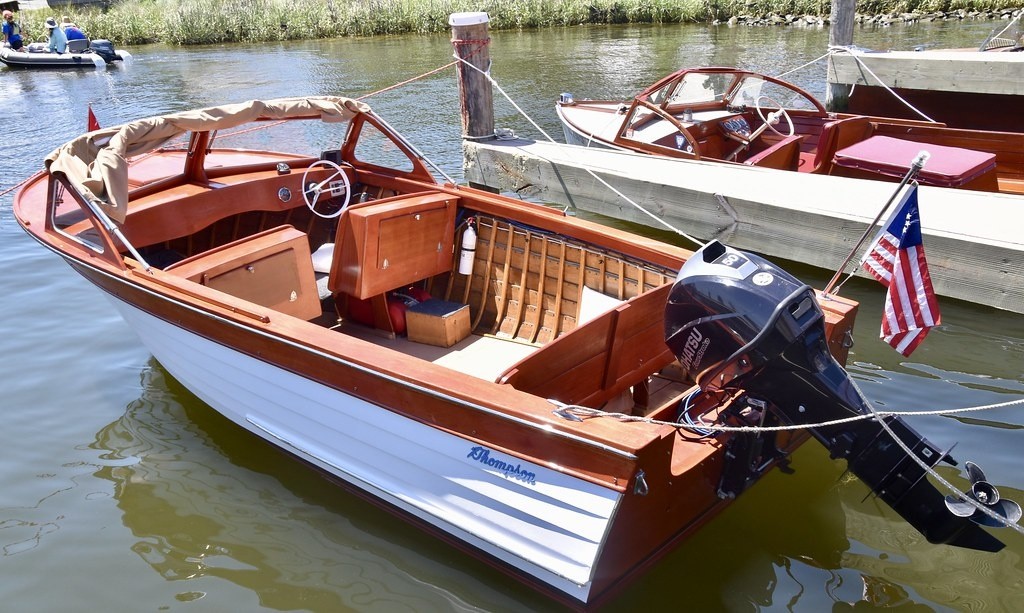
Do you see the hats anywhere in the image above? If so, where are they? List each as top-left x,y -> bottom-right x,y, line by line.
3,10 -> 13,18
60,16 -> 75,26
44,17 -> 58,28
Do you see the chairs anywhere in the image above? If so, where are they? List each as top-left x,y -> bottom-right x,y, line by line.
307,191 -> 459,340
163,223 -> 322,323
744,134 -> 803,171
797,115 -> 873,174
68,39 -> 88,53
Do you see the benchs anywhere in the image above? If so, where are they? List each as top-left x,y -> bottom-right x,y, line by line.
493,281 -> 676,417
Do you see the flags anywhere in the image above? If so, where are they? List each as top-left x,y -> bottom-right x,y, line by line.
860,180 -> 941,358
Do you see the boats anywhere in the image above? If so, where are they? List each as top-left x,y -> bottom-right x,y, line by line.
12,95 -> 1024,611
0,40 -> 125,69
556,65 -> 1024,319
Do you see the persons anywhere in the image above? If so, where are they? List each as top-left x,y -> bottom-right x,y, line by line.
42,17 -> 68,54
1,10 -> 24,52
61,16 -> 86,40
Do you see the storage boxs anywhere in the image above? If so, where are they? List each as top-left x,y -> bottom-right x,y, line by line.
406,298 -> 471,348
